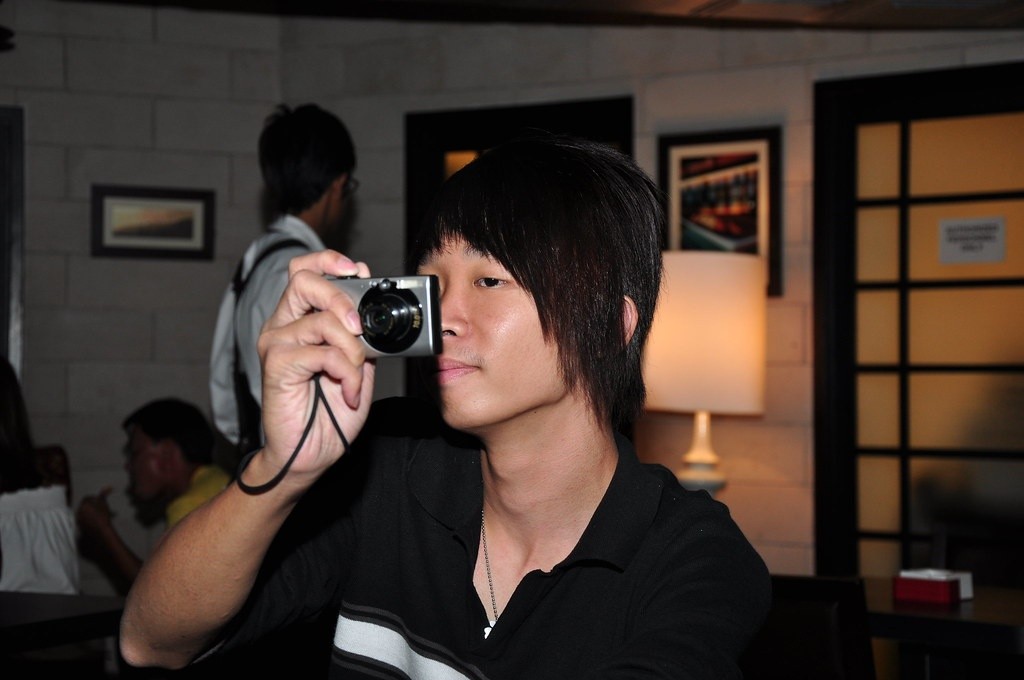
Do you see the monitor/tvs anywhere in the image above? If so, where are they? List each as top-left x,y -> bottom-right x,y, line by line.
403,98 -> 637,276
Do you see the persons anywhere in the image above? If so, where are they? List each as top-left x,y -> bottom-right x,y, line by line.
120,143 -> 776,680
208,102 -> 357,454
78,396 -> 241,597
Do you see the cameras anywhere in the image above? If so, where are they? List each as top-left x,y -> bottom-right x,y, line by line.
312,273 -> 443,360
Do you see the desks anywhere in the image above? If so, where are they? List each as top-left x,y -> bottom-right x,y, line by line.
0,591 -> 125,680
866,573 -> 1024,680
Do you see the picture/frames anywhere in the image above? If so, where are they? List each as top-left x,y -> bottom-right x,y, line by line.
654,124 -> 783,301
90,182 -> 217,262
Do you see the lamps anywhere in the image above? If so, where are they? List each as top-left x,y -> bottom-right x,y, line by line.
639,250 -> 768,491
